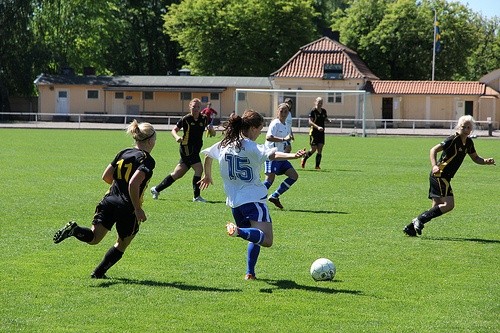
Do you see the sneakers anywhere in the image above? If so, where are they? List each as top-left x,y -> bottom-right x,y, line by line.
53,221 -> 78,244
194,196 -> 207,203
403,226 -> 417,237
412,217 -> 424,235
244,274 -> 257,280
301,158 -> 306,168
268,196 -> 284,209
150,187 -> 160,200
91,272 -> 106,279
315,167 -> 321,169
227,223 -> 238,238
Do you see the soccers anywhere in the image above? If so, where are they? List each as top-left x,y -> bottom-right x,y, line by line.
309,257 -> 337,282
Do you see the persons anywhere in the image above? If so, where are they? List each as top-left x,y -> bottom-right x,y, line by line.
402,115 -> 497,237
150,97 -> 217,203
285,98 -> 294,153
54,120 -> 157,280
263,102 -> 294,210
195,110 -> 308,281
301,96 -> 332,169
199,102 -> 218,138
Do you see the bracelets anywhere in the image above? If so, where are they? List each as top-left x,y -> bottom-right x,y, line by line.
282,136 -> 284,142
